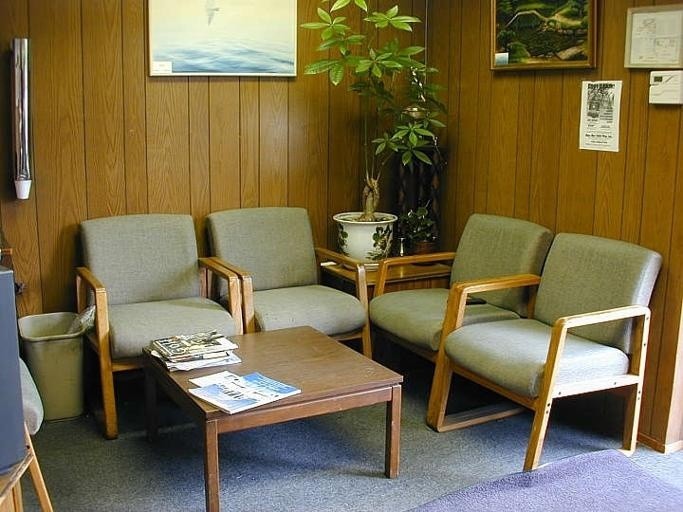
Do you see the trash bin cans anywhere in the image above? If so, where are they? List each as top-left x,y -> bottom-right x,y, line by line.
17,312 -> 86,424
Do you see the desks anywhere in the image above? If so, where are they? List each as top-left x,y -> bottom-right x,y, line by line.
141,326 -> 405,512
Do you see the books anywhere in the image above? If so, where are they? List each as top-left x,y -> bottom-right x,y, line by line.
186,370 -> 301,416
148,329 -> 242,373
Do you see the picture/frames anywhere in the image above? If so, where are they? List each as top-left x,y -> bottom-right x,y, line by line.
147,0 -> 299,77
488,0 -> 600,74
623,2 -> 683,69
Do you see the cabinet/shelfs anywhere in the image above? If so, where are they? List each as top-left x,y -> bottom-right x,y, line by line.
317,260 -> 451,375
